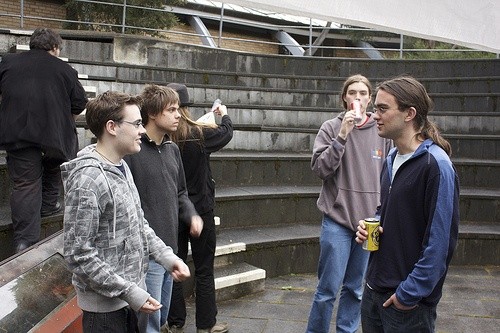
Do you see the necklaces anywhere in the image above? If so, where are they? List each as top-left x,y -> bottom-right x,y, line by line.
94,149 -> 116,164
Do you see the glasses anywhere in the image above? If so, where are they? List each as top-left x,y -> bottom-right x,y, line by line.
373,107 -> 395,111
122,119 -> 143,127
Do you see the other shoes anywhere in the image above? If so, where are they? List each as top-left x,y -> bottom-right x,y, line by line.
197,325 -> 227,331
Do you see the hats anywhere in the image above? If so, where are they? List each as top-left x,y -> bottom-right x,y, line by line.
168,81 -> 193,105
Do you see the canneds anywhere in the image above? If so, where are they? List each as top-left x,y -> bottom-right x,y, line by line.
362,218 -> 380,251
351,100 -> 362,121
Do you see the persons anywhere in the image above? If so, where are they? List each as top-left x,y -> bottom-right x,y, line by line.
304,74 -> 395,333
166,82 -> 233,333
122,84 -> 204,333
59,89 -> 190,333
0,27 -> 89,255
355,77 -> 460,333
